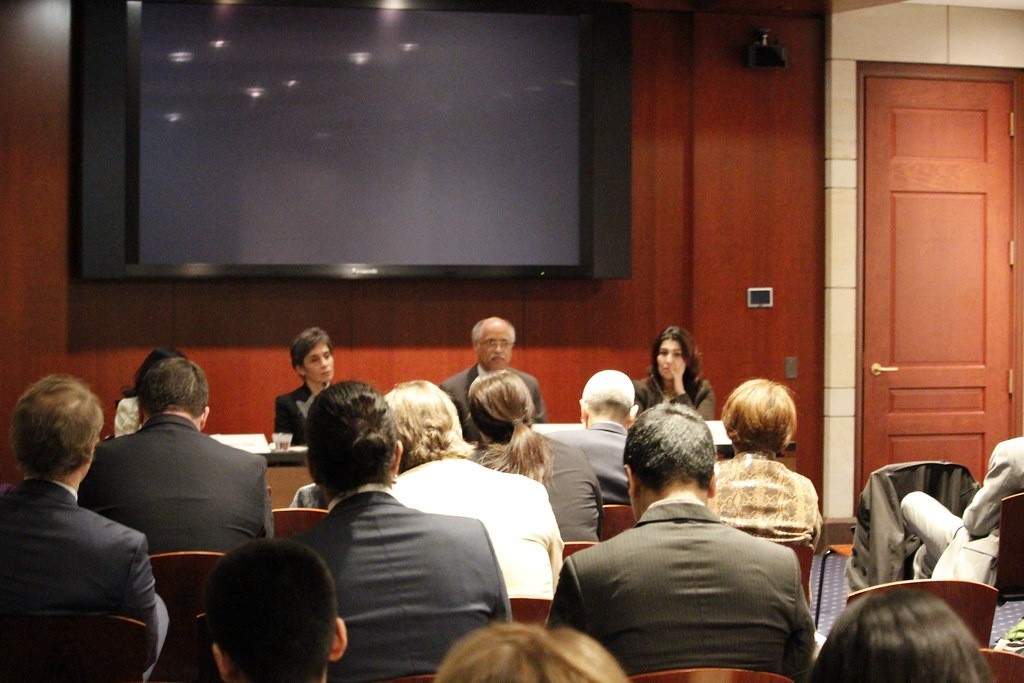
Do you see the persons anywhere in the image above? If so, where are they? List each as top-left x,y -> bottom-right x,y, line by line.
434,317 -> 547,445
704,379 -> 824,548
291,379 -> 515,683
467,370 -> 608,547
77,357 -> 274,683
634,326 -> 716,422
808,589 -> 997,683
542,369 -> 638,506
898,436 -> 1024,596
113,345 -> 189,440
204,537 -> 348,683
0,374 -> 160,683
434,621 -> 629,683
383,381 -> 564,600
273,327 -> 336,446
544,401 -> 816,683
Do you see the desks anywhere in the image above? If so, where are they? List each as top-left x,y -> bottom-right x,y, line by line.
259,449 -> 736,509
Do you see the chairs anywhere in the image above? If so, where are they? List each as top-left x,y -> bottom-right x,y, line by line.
0,457 -> 1024,683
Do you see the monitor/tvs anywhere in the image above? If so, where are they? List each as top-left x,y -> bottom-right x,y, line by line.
67,0 -> 633,279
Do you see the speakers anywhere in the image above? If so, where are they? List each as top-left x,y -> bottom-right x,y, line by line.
745,45 -> 788,68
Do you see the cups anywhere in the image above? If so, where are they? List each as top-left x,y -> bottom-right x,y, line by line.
271,433 -> 293,452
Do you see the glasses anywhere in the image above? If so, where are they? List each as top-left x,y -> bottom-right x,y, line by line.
477,341 -> 515,347
